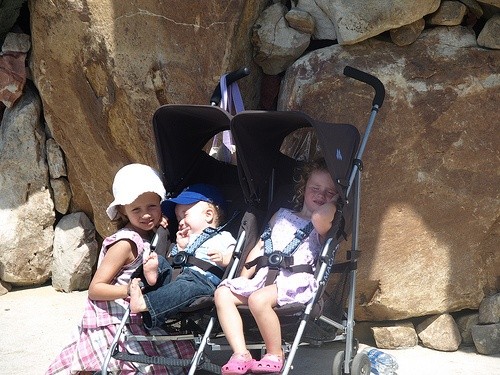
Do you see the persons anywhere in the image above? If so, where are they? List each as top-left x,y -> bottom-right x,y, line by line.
44,163 -> 210,375
130,182 -> 236,330
214,159 -> 341,375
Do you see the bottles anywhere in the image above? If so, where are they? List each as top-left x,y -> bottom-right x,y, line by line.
360,346 -> 399,375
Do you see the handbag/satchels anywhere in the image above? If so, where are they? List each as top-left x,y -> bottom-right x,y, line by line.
209,74 -> 245,165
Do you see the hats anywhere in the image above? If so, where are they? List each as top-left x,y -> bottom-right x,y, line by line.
106,163 -> 166,220
160,184 -> 225,217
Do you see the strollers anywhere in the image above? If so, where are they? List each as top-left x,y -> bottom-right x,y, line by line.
100,66 -> 386,372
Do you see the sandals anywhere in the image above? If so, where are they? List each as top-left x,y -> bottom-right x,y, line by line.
221,351 -> 258,375
251,347 -> 285,373
